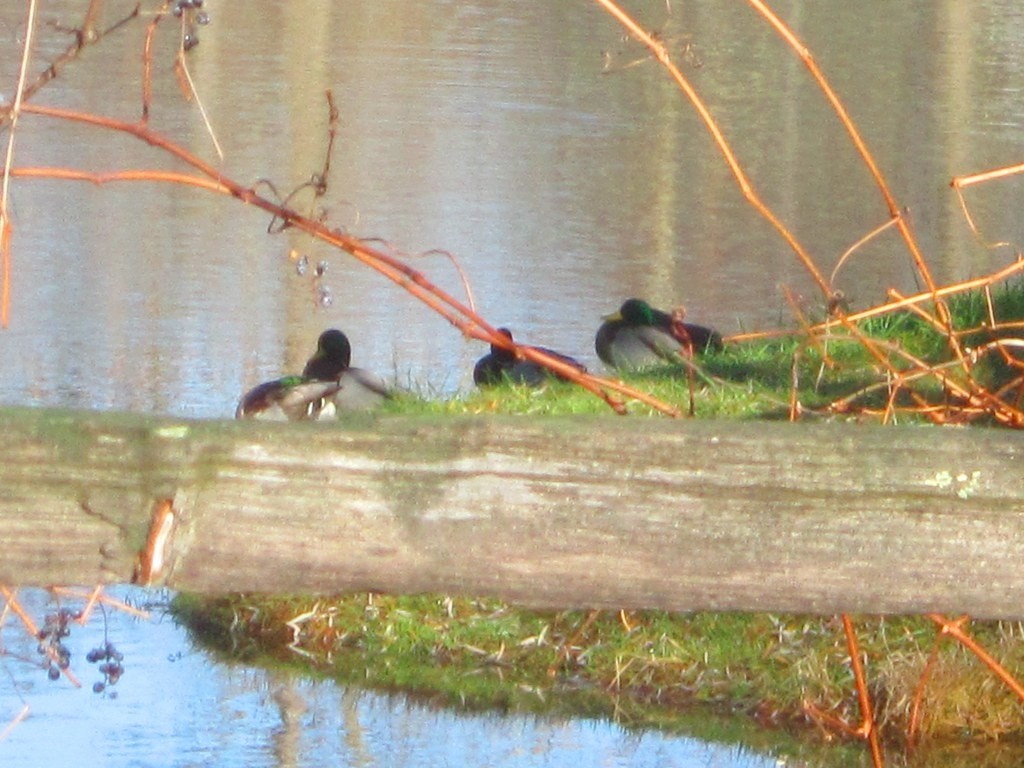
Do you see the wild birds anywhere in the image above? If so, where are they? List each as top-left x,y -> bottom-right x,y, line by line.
298,328 -> 412,418
232,374 -> 340,420
471,327 -> 591,388
593,298 -> 724,375
